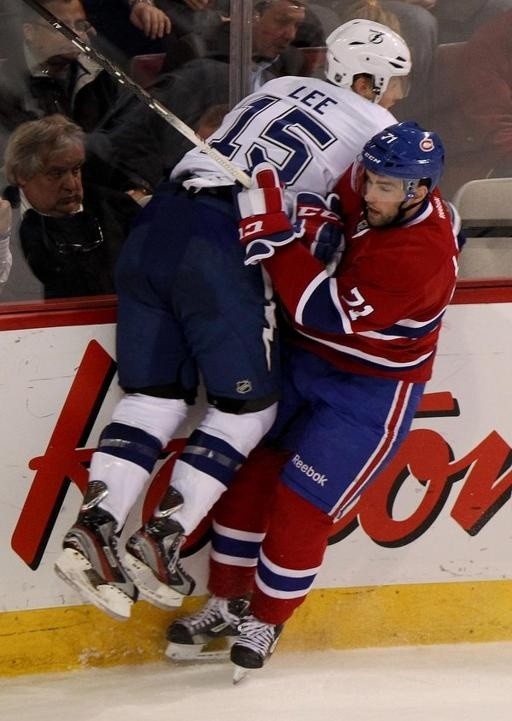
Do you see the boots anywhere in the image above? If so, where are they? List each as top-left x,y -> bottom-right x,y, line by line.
62,478 -> 140,605
124,484 -> 198,598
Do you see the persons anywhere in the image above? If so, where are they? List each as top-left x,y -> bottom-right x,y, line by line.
163,123 -> 459,668
65,17 -> 417,603
1,0 -> 512,304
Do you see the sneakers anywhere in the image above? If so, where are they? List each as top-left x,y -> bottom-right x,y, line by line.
164,593 -> 251,646
229,614 -> 284,670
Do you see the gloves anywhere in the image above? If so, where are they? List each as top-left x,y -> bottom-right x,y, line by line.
287,189 -> 348,278
233,161 -> 303,268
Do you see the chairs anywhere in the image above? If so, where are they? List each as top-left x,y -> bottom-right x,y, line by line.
125,36 -> 512,296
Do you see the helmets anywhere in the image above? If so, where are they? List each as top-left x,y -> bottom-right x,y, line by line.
361,121 -> 446,199
322,18 -> 413,90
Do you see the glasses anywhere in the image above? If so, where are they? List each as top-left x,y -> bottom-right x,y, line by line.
41,212 -> 108,257
28,18 -> 92,35
